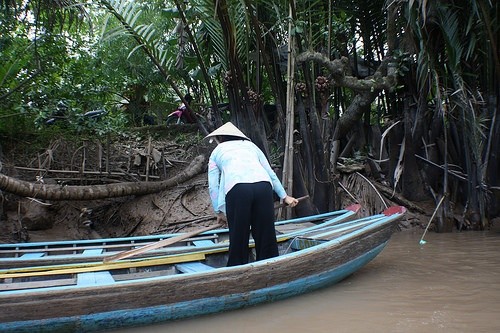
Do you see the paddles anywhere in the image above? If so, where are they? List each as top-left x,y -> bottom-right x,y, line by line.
99,195 -> 308,262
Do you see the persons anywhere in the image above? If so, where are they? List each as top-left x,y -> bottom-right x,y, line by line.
177,93 -> 192,125
203,122 -> 298,266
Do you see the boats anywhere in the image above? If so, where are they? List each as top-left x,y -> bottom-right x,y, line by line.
0,201 -> 361,269
0,204 -> 407,333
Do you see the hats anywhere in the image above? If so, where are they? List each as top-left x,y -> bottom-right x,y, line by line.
204,121 -> 251,141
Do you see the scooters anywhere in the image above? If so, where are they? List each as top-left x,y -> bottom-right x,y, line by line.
47,97 -> 109,131
165,95 -> 230,126
